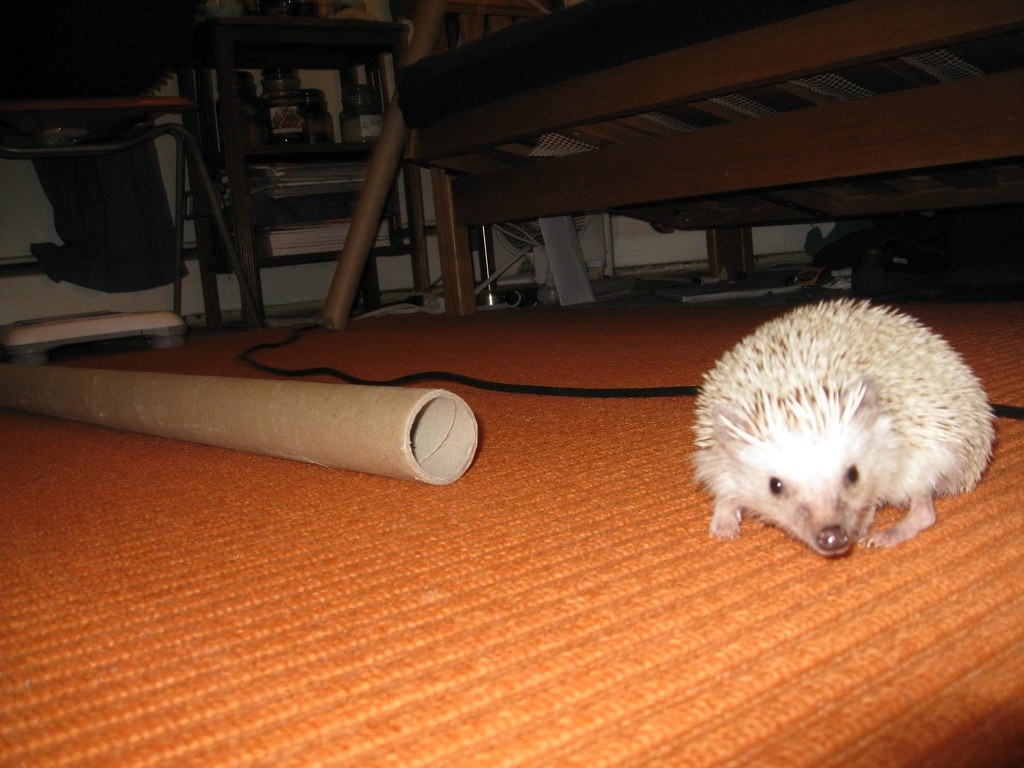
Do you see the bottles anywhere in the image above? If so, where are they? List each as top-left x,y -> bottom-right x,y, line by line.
213,69 -> 382,145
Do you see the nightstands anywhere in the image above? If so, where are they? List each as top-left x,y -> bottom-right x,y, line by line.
190,16 -> 429,338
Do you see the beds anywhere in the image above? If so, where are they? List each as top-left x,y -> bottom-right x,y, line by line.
407,0 -> 1024,318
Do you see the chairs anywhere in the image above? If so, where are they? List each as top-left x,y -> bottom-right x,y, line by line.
0,97 -> 265,333
